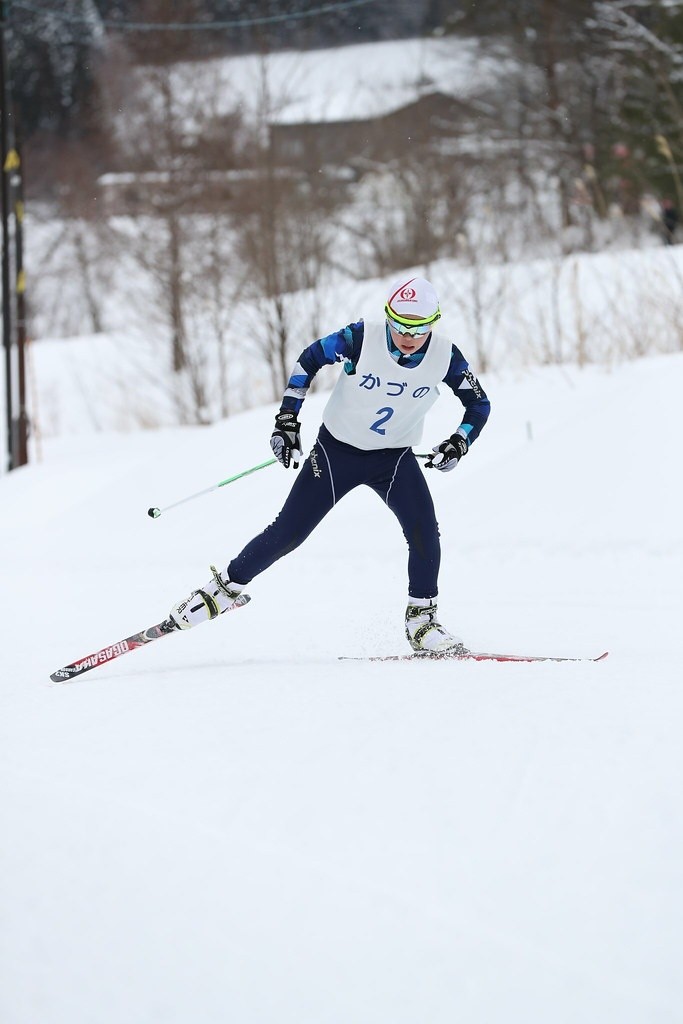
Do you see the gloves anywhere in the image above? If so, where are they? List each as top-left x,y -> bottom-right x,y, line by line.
425,433 -> 468,472
269,409 -> 303,469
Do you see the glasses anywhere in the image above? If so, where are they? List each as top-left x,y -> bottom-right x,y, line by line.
387,315 -> 432,339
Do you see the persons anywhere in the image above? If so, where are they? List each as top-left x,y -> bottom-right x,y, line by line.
164,277 -> 491,654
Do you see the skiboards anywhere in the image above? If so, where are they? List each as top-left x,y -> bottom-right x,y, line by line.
48,592 -> 610,684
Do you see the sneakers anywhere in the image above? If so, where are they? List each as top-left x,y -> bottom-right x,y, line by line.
169,560 -> 250,631
405,593 -> 465,654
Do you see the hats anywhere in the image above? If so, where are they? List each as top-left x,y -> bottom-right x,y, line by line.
388,274 -> 439,318
